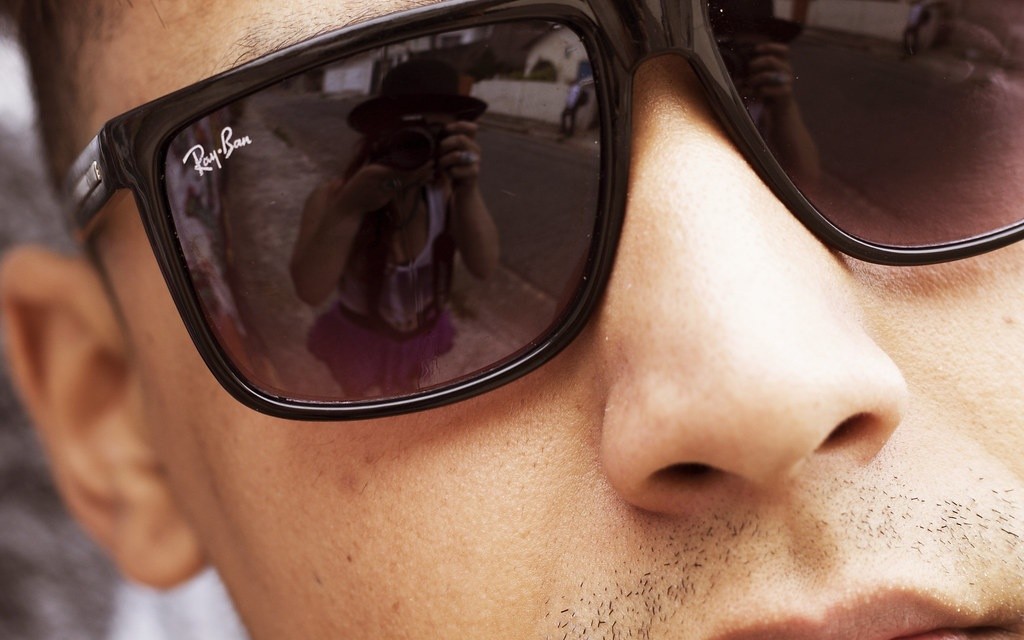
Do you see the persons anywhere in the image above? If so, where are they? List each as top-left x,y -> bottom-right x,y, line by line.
555,79 -> 590,127
292,57 -> 498,396
709,0 -> 821,191
0,0 -> 1024,640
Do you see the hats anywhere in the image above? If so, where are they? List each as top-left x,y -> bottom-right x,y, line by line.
706,3 -> 801,49
345,56 -> 489,140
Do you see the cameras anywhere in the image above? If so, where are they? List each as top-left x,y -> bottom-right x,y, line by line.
712,38 -> 755,86
359,121 -> 451,173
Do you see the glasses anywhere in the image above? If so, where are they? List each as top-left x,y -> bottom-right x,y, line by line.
66,1 -> 1024,424
